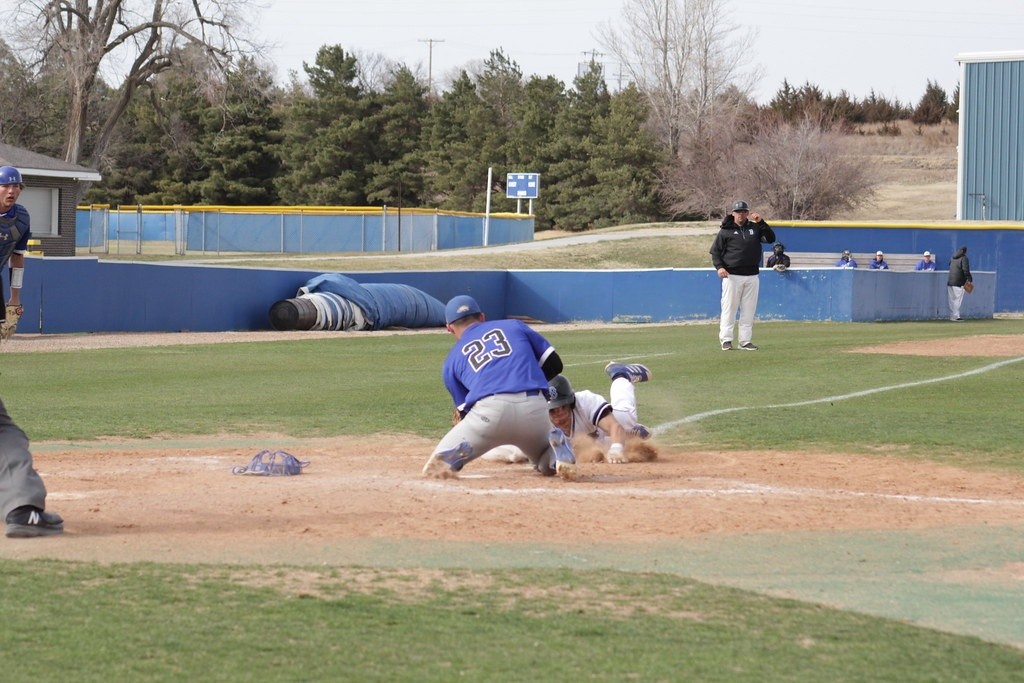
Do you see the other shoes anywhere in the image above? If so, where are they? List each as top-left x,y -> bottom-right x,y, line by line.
423,441 -> 475,479
955,318 -> 965,321
548,428 -> 579,482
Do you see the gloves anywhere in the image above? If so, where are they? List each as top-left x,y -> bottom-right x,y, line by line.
605,443 -> 628,464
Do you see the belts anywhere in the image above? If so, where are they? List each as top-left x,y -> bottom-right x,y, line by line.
493,386 -> 542,395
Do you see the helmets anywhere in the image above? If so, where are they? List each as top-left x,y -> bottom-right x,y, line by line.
772,242 -> 786,249
548,375 -> 575,409
445,295 -> 482,324
842,250 -> 851,260
0,166 -> 23,184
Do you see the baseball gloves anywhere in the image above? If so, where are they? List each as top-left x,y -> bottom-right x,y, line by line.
1,305 -> 23,338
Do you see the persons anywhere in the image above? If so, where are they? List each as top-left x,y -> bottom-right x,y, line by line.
766,241 -> 790,272
546,362 -> 654,462
0,166 -> 30,342
836,250 -> 857,268
868,251 -> 888,269
423,294 -> 577,481
0,398 -> 64,538
708,200 -> 775,350
914,251 -> 937,271
947,246 -> 973,321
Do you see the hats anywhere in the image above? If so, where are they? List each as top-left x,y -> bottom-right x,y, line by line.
924,251 -> 930,257
876,251 -> 883,256
733,201 -> 750,211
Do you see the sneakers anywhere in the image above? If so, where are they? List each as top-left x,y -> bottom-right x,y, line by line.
722,341 -> 733,351
5,505 -> 64,537
738,342 -> 758,350
604,362 -> 654,383
626,423 -> 653,441
